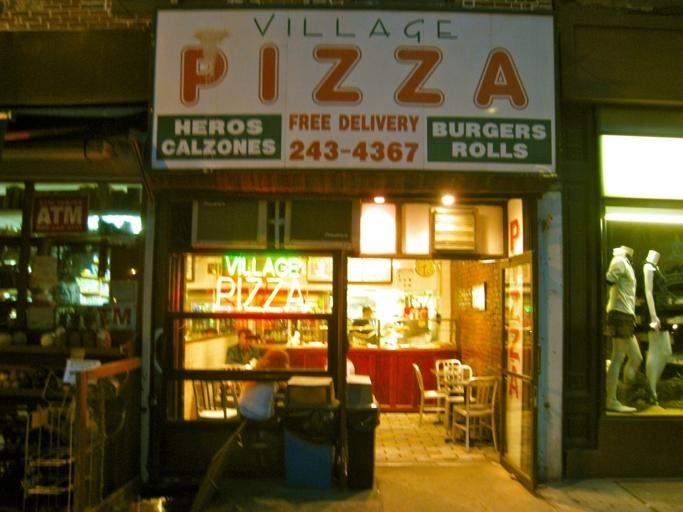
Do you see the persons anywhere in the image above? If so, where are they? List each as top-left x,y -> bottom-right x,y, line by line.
351,305 -> 379,346
225,328 -> 260,365
606,246 -> 642,414
236,346 -> 290,423
643,250 -> 673,407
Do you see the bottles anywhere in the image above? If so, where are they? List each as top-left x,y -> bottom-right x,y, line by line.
193,305 -> 209,332
265,329 -> 287,344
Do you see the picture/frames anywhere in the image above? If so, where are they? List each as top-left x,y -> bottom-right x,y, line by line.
186,252 -> 195,283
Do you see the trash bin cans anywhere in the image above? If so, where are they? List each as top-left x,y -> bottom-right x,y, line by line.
343,374 -> 381,491
274,375 -> 341,492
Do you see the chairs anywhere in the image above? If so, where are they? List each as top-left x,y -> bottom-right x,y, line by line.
436,359 -> 464,424
444,364 -> 476,441
192,378 -> 241,424
452,376 -> 502,452
412,361 -> 449,425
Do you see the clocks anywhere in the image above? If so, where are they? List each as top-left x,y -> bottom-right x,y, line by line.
415,259 -> 435,277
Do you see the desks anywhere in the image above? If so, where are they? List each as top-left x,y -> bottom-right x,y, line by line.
431,368 -> 451,377
450,381 -> 493,404
